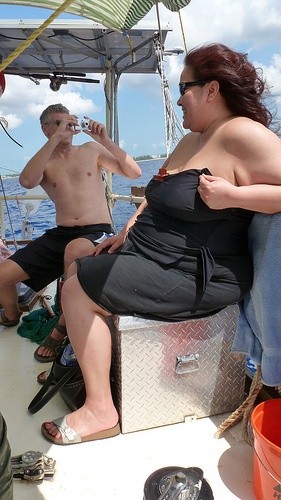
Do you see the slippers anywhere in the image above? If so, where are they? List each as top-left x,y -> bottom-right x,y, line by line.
38,368 -> 51,383
41,415 -> 120,445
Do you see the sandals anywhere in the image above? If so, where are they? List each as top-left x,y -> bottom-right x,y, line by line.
0,309 -> 20,326
34,323 -> 67,363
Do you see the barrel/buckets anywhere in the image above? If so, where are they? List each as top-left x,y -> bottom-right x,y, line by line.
250,397 -> 281,500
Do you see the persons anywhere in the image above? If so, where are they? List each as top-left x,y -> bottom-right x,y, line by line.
37,43 -> 281,444
0,103 -> 141,362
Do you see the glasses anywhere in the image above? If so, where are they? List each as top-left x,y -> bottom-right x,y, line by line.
46,119 -> 61,125
179,81 -> 206,95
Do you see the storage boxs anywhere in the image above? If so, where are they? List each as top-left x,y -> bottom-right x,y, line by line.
105,304 -> 245,434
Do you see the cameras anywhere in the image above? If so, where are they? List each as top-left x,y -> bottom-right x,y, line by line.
72,118 -> 90,131
